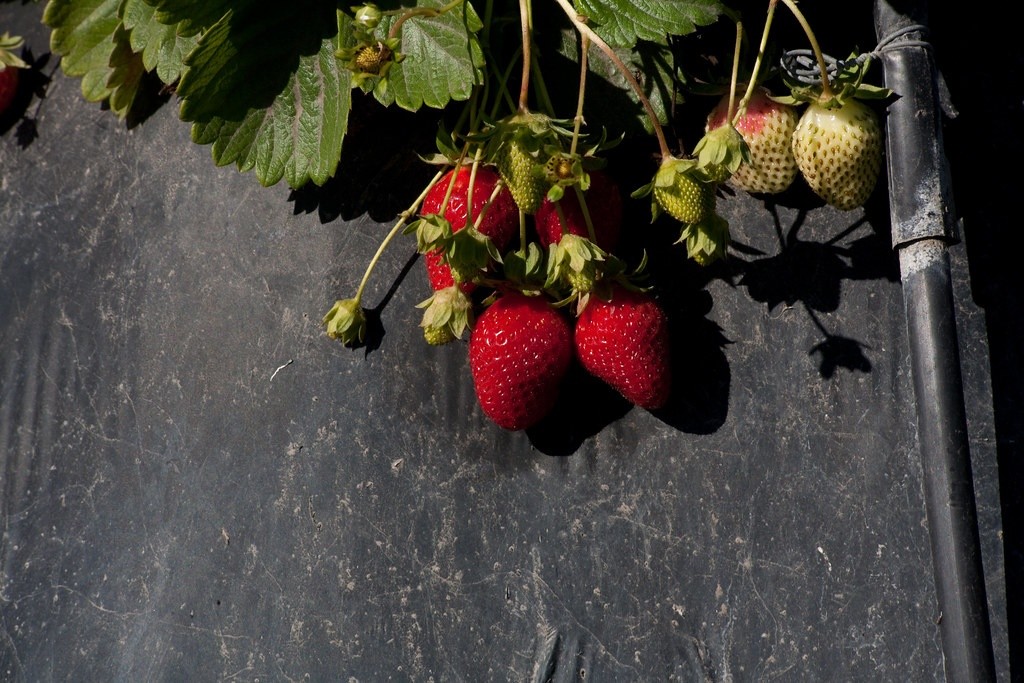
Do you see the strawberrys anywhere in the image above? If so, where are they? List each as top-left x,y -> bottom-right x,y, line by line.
322,42 -> 880,428
0,32 -> 33,109
